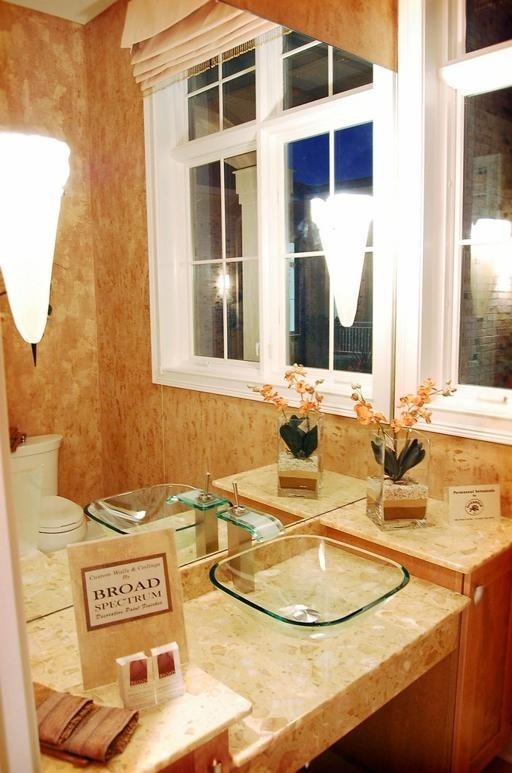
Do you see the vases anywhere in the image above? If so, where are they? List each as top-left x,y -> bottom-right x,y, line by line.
276,412 -> 321,499
366,428 -> 432,531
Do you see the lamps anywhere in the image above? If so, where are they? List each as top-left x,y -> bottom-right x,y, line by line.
309,192 -> 374,328
0,132 -> 71,366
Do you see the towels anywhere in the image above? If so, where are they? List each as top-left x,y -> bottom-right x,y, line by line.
31,682 -> 139,768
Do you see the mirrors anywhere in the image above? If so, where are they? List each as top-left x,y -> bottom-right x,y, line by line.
0,0 -> 398,621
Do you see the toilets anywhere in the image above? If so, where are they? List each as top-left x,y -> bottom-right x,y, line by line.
11,433 -> 87,564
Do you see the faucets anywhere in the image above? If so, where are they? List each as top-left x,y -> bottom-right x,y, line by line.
173,469 -> 228,548
217,480 -> 285,593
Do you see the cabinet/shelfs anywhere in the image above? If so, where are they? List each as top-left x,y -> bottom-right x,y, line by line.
322,528 -> 512,773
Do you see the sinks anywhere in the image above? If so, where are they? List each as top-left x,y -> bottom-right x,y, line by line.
83,483 -> 204,535
210,534 -> 410,628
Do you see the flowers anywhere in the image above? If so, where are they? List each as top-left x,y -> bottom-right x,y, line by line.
350,377 -> 457,485
246,363 -> 325,461
